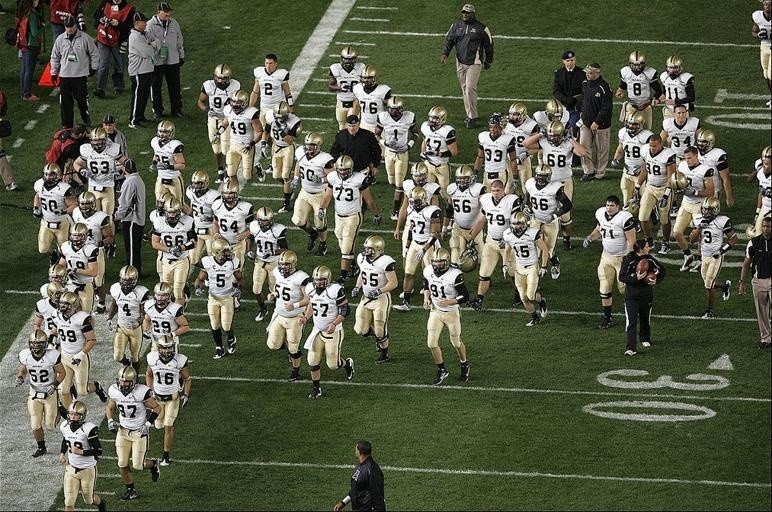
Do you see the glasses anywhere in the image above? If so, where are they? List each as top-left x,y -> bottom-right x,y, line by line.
566,60 -> 573,62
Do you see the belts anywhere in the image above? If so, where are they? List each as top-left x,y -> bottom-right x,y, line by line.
415,240 -> 427,245
460,226 -> 471,231
337,214 -> 349,217
275,145 -> 287,153
364,297 -> 378,306
492,237 -> 502,241
429,161 -> 441,167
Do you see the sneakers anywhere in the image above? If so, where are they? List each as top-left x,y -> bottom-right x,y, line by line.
347,265 -> 359,278
563,235 -> 570,250
98,499 -> 106,512
760,342 -> 768,349
266,165 -> 272,173
641,342 -> 650,347
658,244 -> 672,255
539,298 -> 547,317
432,369 -> 449,385
392,301 -> 411,311
213,346 -> 225,359
723,280 -> 731,301
93,380 -> 108,402
646,243 -> 655,249
331,278 -> 345,286
22,95 -> 39,101
467,119 -> 479,128
152,459 -> 160,482
171,112 -> 184,117
94,91 -> 105,98
215,169 -> 224,184
465,298 -> 481,311
314,243 -> 327,257
70,386 -> 77,398
58,402 -> 66,419
420,288 -> 425,294
690,260 -> 701,272
288,374 -> 301,382
277,203 -> 290,214
390,208 -> 399,221
128,124 -> 141,129
511,299 -> 522,307
308,230 -> 318,251
160,456 -> 171,466
32,447 -> 46,457
702,310 -> 715,319
255,164 -> 266,182
551,258 -> 561,280
526,317 -> 540,327
464,117 -> 469,123
6,182 -> 16,190
345,358 -> 354,380
599,318 -> 615,329
183,282 -> 191,302
227,337 -> 237,354
308,387 -> 321,398
625,349 -> 637,357
458,364 -> 470,383
399,288 -> 415,298
580,174 -> 593,180
119,489 -> 137,502
152,111 -> 161,119
375,356 -> 390,365
139,119 -> 149,126
254,310 -> 268,321
680,254 -> 694,271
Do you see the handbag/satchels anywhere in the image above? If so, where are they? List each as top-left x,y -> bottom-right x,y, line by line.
6,28 -> 17,45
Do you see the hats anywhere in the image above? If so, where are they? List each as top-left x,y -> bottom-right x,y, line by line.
64,16 -> 75,26
103,115 -> 115,123
133,12 -> 148,21
582,64 -> 600,72
562,51 -> 575,59
346,115 -> 358,124
460,4 -> 475,13
124,159 -> 136,173
157,2 -> 173,11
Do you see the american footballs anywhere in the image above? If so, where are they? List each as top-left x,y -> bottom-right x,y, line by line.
636,259 -> 649,274
643,272 -> 655,285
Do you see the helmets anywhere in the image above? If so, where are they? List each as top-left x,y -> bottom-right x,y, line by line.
697,129 -> 715,155
43,163 -> 62,186
408,186 -> 427,209
59,292 -> 80,318
666,55 -> 683,77
626,111 -> 645,134
117,366 -> 137,394
156,192 -> 173,216
340,47 -> 357,72
211,239 -> 234,261
545,99 -> 563,121
455,165 -> 475,190
762,145 -> 771,160
47,281 -> 63,302
387,96 -> 404,120
213,64 -> 231,84
458,246 -> 477,273
231,89 -> 249,114
746,226 -> 755,240
428,105 -> 447,128
336,155 -> 354,180
410,162 -> 428,185
165,197 -> 181,225
534,164 -> 552,186
157,335 -> 175,361
431,248 -> 450,272
361,65 -> 376,87
278,250 -> 297,277
547,120 -> 564,146
509,102 -> 528,123
67,401 -> 87,425
362,235 -> 385,262
49,264 -> 68,283
70,223 -> 88,247
157,120 -> 175,142
29,329 -> 48,357
119,265 -> 138,290
221,181 -> 241,207
273,101 -> 289,124
78,192 -> 97,219
312,266 -> 331,289
256,207 -> 274,231
628,51 -> 646,72
488,111 -> 508,130
305,132 -> 323,157
700,197 -> 721,222
90,127 -> 107,152
153,282 -> 171,309
672,171 -> 688,194
191,171 -> 209,192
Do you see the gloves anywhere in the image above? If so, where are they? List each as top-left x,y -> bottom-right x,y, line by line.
259,149 -> 268,159
148,161 -> 157,171
466,240 -> 475,248
67,269 -> 79,281
312,175 -> 322,183
631,187 -> 639,203
340,83 -> 349,93
388,140 -> 398,150
180,395 -> 188,408
54,209 -> 67,215
368,289 -> 382,298
583,238 -> 591,248
543,214 -> 555,224
107,320 -> 114,330
659,194 -> 668,208
51,75 -> 56,82
88,69 -> 96,77
208,110 -> 216,117
351,287 -> 360,298
156,161 -> 168,170
96,240 -> 104,247
128,321 -> 139,329
760,188 -> 770,196
499,239 -> 505,249
685,184 -> 695,196
15,376 -> 24,386
108,418 -> 119,433
373,214 -> 382,226
502,266 -> 508,278
757,29 -> 767,40
519,152 -> 527,162
247,250 -> 254,260
45,383 -> 57,395
79,168 -> 92,178
522,207 -> 532,217
212,135 -> 219,145
540,128 -> 547,137
318,208 -> 324,221
699,218 -> 709,228
139,421 -> 151,435
200,214 -> 212,223
241,140 -> 256,154
711,250 -> 725,259
611,159 -> 620,167
231,285 -> 243,300
165,332 -> 173,338
415,249 -> 426,261
290,176 -> 299,189
262,249 -> 275,259
397,144 -> 409,154
484,62 -> 491,70
511,180 -> 519,191
33,206 -> 41,218
179,58 -> 184,66
418,152 -> 427,162
167,245 -> 182,257
195,288 -> 204,297
538,266 -> 547,279
71,351 -> 85,366
564,129 -> 570,140
142,330 -> 152,339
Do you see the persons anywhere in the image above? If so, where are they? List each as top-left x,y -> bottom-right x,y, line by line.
7,0 -> 772,512
